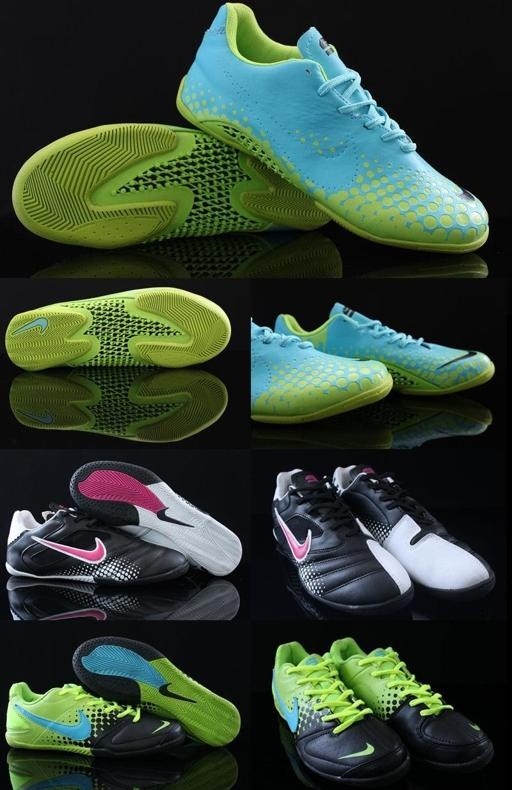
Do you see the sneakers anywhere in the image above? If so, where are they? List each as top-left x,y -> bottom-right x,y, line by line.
69,461 -> 243,577
6,287 -> 231,369
254,398 -> 493,450
269,469 -> 414,616
274,304 -> 496,394
5,681 -> 185,754
12,121 -> 330,250
10,373 -> 228,442
6,753 -> 241,790
6,505 -> 188,582
330,637 -> 494,773
249,319 -> 392,423
177,2 -> 491,253
72,637 -> 242,747
329,466 -> 496,598
7,584 -> 240,621
271,642 -> 410,783
